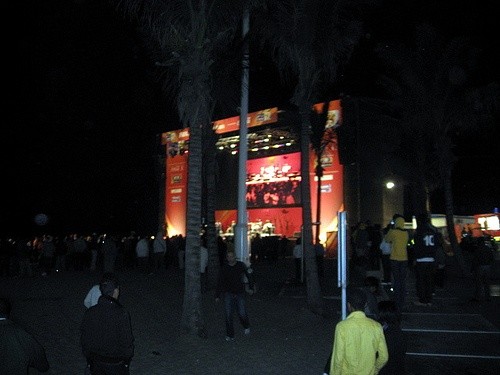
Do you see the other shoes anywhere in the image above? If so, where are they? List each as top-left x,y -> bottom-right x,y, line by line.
381,280 -> 391,283
226,337 -> 234,342
413,300 -> 431,307
245,329 -> 250,335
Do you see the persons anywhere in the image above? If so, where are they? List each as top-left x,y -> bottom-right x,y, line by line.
323,288 -> 407,375
79,272 -> 136,375
0,296 -> 50,375
346,213 -> 498,317
216,249 -> 256,343
0,231 -> 325,300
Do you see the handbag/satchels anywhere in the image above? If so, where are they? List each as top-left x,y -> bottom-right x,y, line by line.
430,228 -> 449,265
236,262 -> 254,295
